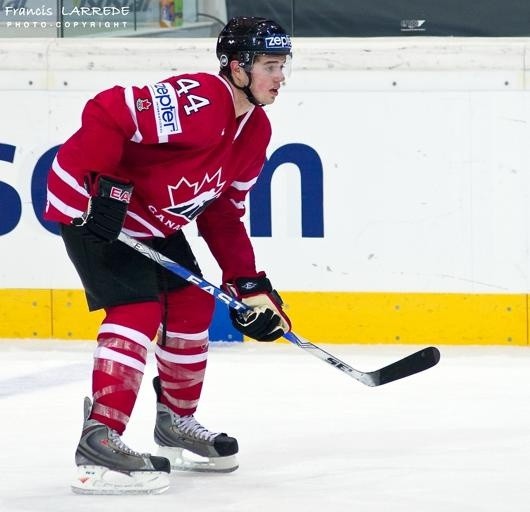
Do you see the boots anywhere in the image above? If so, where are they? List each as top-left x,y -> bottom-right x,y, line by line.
151,376 -> 239,458
74,394 -> 171,475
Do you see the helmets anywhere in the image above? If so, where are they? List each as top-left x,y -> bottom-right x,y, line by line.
215,16 -> 292,61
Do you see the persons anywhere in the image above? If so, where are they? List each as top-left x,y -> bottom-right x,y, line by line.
44,17 -> 291,473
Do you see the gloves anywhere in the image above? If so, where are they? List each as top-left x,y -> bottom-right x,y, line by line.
218,269 -> 293,341
65,172 -> 133,242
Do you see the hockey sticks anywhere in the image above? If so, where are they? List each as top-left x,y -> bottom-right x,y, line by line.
82,210 -> 440,387
